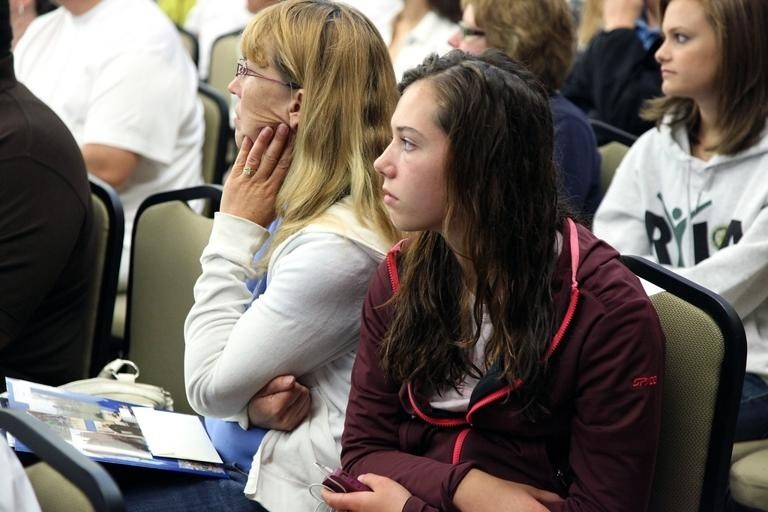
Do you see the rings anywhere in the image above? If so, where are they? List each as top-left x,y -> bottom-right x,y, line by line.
243,167 -> 257,176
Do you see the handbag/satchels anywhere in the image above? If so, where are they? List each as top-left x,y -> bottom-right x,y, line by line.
59,357 -> 174,412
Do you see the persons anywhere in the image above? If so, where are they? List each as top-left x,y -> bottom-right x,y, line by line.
448,2 -> 603,231
183,3 -> 414,512
180,2 -> 278,81
590,3 -> 768,443
338,2 -> 462,84
3,2 -> 95,402
319,51 -> 681,512
13,2 -> 207,294
563,3 -> 668,148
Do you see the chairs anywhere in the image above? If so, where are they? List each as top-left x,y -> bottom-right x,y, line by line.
119,182 -> 222,412
204,25 -> 246,165
588,117 -> 638,198
176,23 -> 200,67
112,78 -> 230,342
76,173 -> 124,384
616,253 -> 748,512
0,404 -> 127,512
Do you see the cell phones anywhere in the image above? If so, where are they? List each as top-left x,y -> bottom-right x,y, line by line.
323,469 -> 372,493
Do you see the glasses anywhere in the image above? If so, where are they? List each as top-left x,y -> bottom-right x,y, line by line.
235,58 -> 301,90
458,21 -> 485,38
